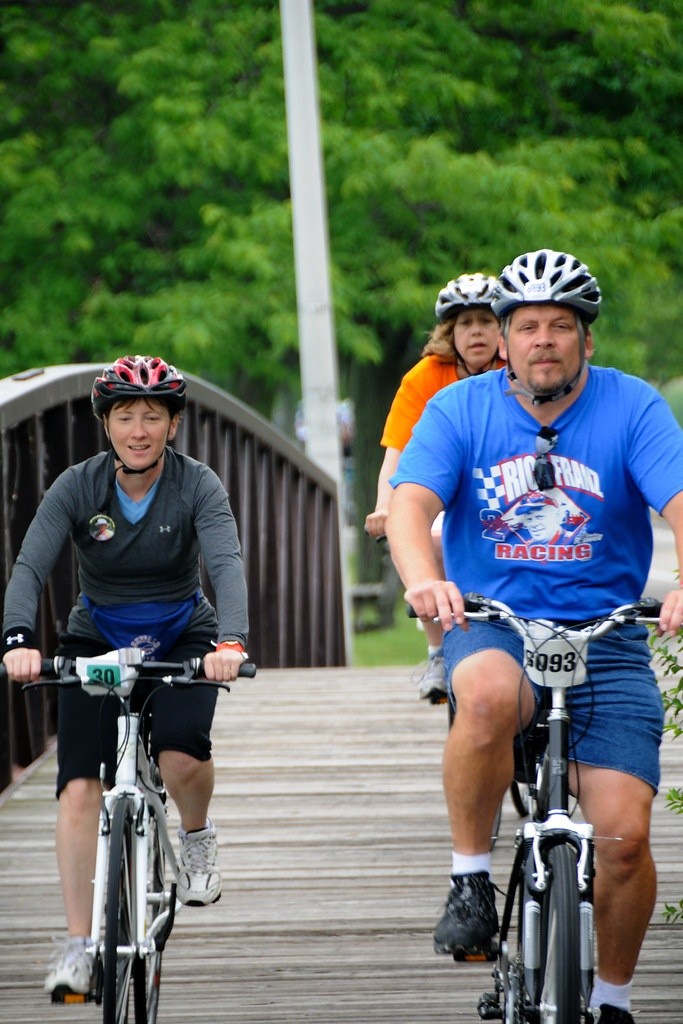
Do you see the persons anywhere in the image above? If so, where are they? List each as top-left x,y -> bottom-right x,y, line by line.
381,249 -> 683,1024
363,273 -> 506,702
3,355 -> 252,1000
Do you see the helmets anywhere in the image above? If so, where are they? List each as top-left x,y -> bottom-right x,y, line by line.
489,247 -> 602,323
91,352 -> 187,421
435,272 -> 500,318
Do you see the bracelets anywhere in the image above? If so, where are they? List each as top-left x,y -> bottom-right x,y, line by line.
214,641 -> 244,652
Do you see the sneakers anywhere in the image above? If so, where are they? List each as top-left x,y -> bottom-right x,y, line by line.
581,1002 -> 635,1024
45,934 -> 97,1002
174,816 -> 224,907
430,871 -> 503,955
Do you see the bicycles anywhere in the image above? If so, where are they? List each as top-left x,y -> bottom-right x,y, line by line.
405,592 -> 683,1023
1,655 -> 259,1024
364,522 -> 536,854
